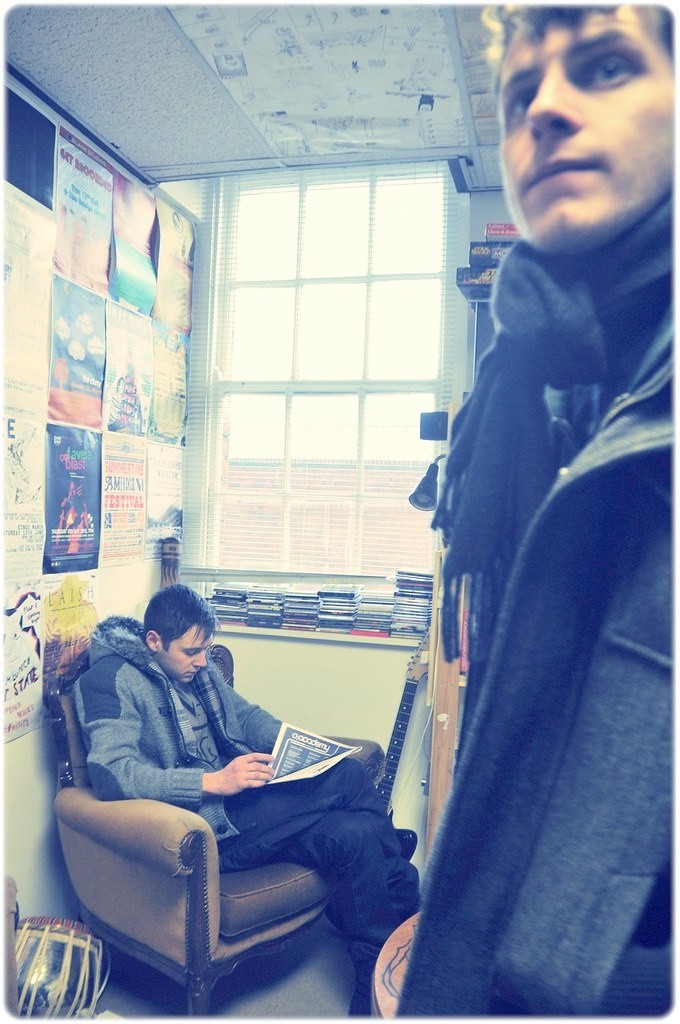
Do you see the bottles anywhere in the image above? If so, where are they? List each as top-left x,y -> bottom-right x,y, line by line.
161,538 -> 181,589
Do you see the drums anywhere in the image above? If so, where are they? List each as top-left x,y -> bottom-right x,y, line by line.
14,914 -> 103,1019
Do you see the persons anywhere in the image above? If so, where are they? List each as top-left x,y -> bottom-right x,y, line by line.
73,584 -> 419,1016
397,7 -> 673,1016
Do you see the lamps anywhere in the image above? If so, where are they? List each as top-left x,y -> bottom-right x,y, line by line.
410,455 -> 447,511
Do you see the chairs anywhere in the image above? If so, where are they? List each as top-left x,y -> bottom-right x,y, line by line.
43,646 -> 384,1017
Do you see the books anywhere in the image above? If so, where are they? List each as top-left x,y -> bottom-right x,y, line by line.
212,569 -> 435,639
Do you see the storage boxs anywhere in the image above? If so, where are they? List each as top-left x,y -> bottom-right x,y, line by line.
458,225 -> 521,311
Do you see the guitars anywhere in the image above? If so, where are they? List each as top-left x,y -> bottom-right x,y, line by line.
376,626 -> 434,860
